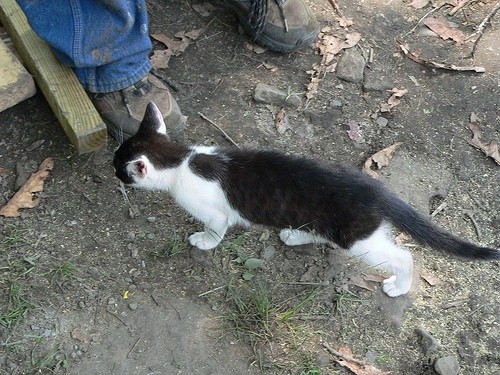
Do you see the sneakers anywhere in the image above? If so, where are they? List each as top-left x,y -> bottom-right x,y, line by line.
85,66 -> 185,145
221,0 -> 321,54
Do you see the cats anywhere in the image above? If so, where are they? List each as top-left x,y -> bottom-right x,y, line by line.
112,101 -> 500,298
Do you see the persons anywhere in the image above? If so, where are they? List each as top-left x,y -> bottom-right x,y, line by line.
20,1 -> 320,142
